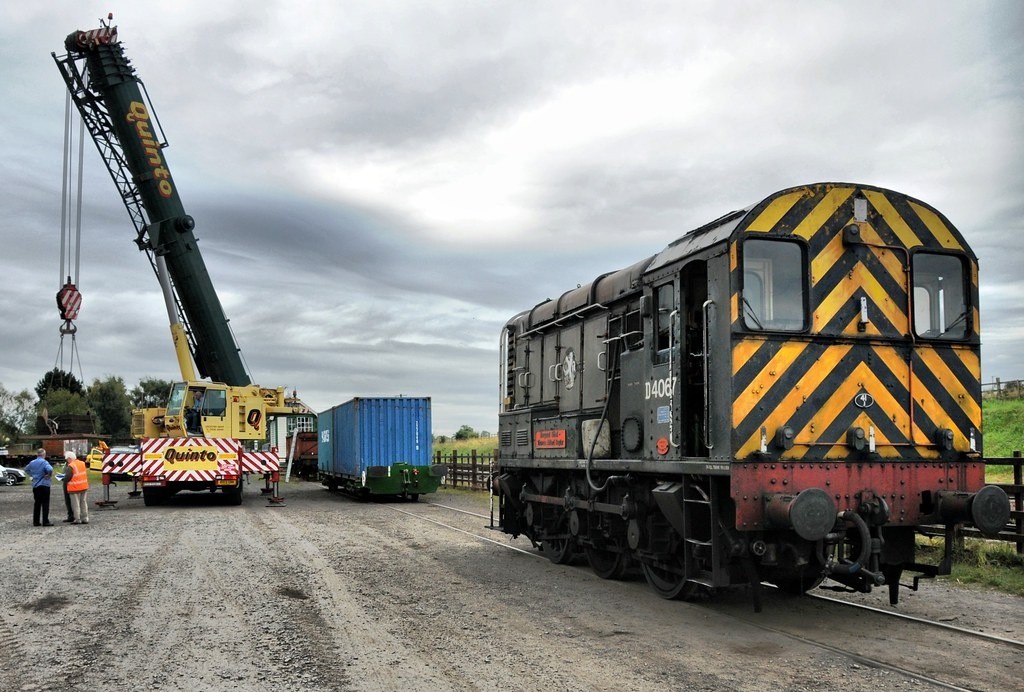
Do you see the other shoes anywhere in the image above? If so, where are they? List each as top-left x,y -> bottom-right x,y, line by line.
70,521 -> 81,525
81,520 -> 88,524
42,523 -> 54,526
62,519 -> 74,522
33,522 -> 42,526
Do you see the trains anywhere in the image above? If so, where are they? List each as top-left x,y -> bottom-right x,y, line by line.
486,181 -> 1012,603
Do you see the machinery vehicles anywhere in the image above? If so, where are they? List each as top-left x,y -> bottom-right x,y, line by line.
48,11 -> 309,507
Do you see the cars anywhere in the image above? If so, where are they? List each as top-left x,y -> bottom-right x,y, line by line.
0,464 -> 27,487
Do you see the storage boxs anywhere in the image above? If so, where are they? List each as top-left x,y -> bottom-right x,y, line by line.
35,413 -> 97,434
42,438 -> 88,456
285,395 -> 433,481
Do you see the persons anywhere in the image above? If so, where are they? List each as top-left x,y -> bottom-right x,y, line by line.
186,391 -> 206,433
61,452 -> 89,524
24,448 -> 54,527
62,451 -> 81,523
